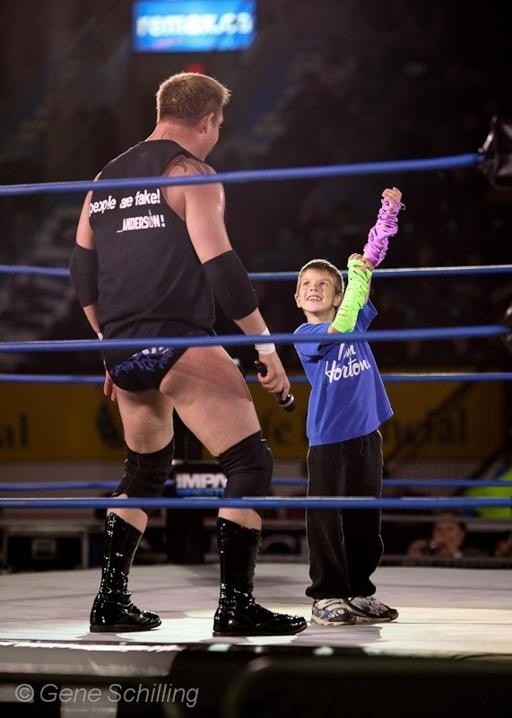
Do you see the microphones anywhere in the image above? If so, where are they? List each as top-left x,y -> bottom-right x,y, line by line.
253,359 -> 296,414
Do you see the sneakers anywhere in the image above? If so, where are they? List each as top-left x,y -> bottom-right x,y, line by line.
310,595 -> 399,624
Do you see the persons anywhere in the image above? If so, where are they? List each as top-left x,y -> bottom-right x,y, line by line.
291,181 -> 406,630
2,488 -> 512,718
67,70 -> 311,639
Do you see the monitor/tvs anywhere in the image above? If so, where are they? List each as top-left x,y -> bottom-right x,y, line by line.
128,1 -> 260,56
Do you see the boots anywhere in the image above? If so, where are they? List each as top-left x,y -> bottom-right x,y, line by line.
88,511 -> 161,632
212,515 -> 307,638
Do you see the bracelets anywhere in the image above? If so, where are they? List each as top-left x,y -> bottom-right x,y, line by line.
254,328 -> 276,357
95,333 -> 109,365
